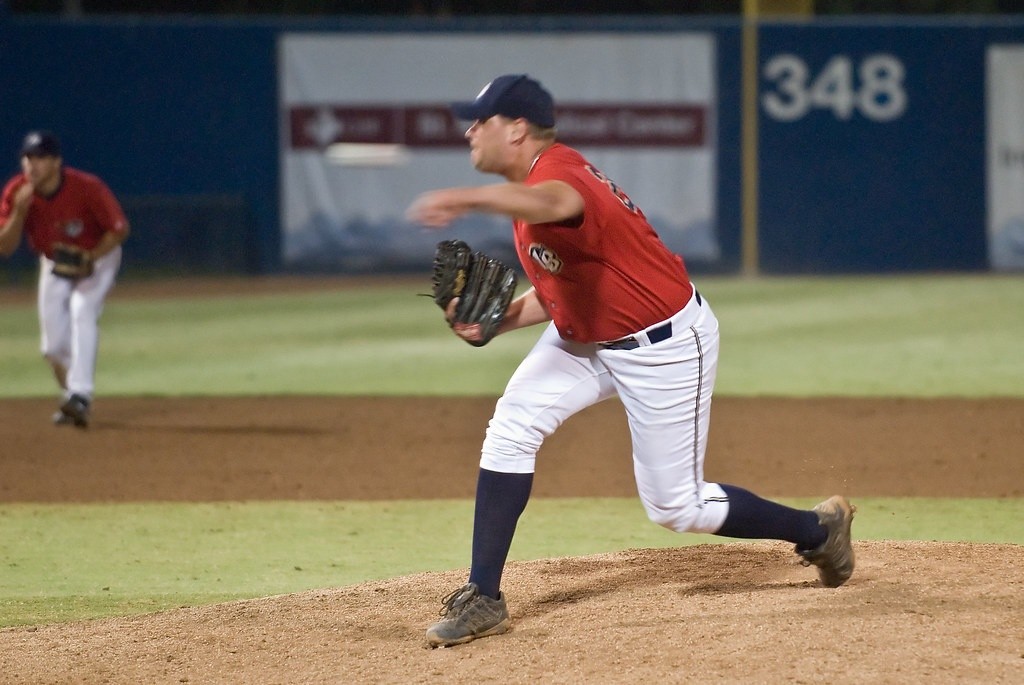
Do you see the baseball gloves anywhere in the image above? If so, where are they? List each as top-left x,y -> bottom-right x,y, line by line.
425,240 -> 517,349
49,241 -> 93,281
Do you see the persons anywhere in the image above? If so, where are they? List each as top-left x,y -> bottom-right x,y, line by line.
404,74 -> 856,647
1,130 -> 128,428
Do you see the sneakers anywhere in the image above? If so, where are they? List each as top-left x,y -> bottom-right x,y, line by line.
427,583 -> 509,648
795,495 -> 856,587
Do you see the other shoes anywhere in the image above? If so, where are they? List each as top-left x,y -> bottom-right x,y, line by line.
60,392 -> 90,425
54,412 -> 73,426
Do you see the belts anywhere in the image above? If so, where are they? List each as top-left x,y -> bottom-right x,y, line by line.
599,291 -> 701,351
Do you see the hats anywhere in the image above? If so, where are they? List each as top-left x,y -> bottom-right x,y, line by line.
18,130 -> 62,158
451,72 -> 555,129
